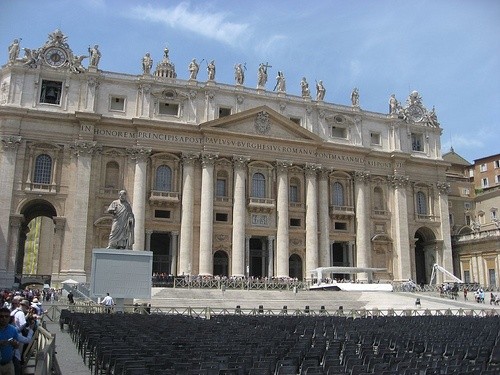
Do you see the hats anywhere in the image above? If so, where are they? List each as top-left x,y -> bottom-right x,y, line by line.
20,300 -> 30,307
31,299 -> 40,305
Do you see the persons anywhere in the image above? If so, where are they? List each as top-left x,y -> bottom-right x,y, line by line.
317,80 -> 326,101
99,292 -> 114,314
473,288 -> 485,304
234,63 -> 244,84
438,283 -> 459,300
188,58 -> 200,79
258,63 -> 268,87
489,293 -> 500,306
351,88 -> 360,106
463,287 -> 468,301
141,53 -> 153,74
277,71 -> 286,92
8,38 -> 20,60
0,288 -> 62,375
106,189 -> 135,250
88,44 -> 102,68
67,289 -> 74,311
389,93 -> 398,114
300,76 -> 311,96
206,60 -> 216,80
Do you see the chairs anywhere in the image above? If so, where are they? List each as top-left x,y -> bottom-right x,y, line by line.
58,313 -> 500,375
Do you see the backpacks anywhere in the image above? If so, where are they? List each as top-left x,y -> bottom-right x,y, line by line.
9,311 -> 19,326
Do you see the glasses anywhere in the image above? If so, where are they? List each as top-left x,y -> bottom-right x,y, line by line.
0,315 -> 9,319
